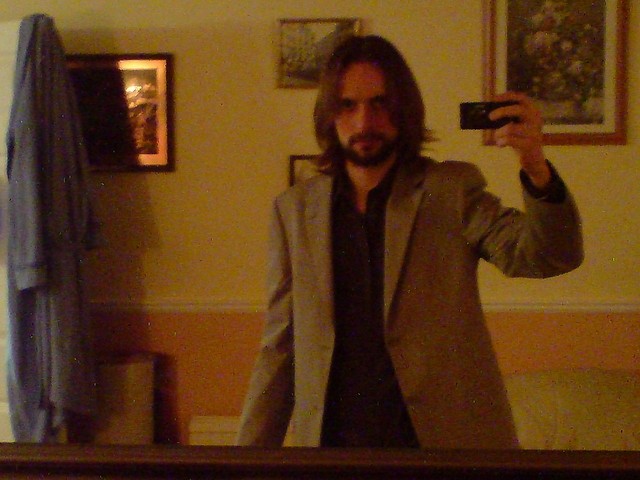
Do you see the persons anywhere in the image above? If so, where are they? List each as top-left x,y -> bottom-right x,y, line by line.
235,35 -> 583,450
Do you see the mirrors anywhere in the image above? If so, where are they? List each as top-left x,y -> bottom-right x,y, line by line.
0,0 -> 640,480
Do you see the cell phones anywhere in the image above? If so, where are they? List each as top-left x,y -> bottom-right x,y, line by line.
459,101 -> 519,130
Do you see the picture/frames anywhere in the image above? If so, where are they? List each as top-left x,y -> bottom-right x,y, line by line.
63,52 -> 178,174
275,16 -> 364,91
288,153 -> 329,188
481,0 -> 630,150
